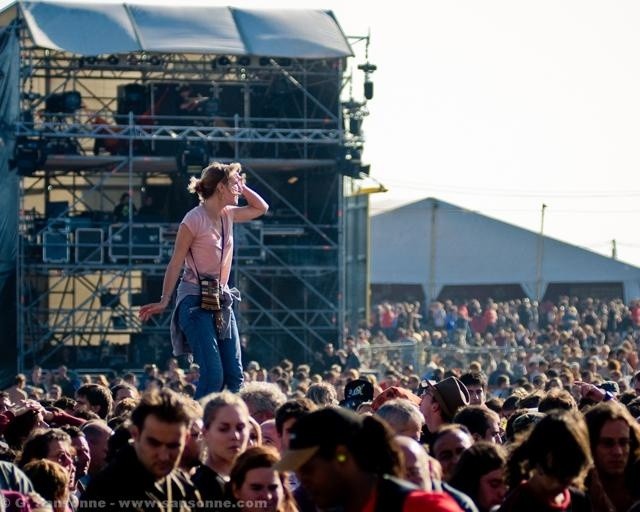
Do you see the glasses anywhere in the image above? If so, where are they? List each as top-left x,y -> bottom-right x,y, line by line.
145,436 -> 182,450
47,450 -> 72,463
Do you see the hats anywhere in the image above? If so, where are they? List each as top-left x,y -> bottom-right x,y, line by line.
424,376 -> 471,417
339,379 -> 374,411
268,406 -> 359,474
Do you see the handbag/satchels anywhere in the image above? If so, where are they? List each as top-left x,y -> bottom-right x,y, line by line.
198,277 -> 223,312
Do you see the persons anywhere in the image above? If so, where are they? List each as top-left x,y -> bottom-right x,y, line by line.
0,296 -> 640,511
138,162 -> 269,400
113,192 -> 137,222
175,83 -> 199,116
